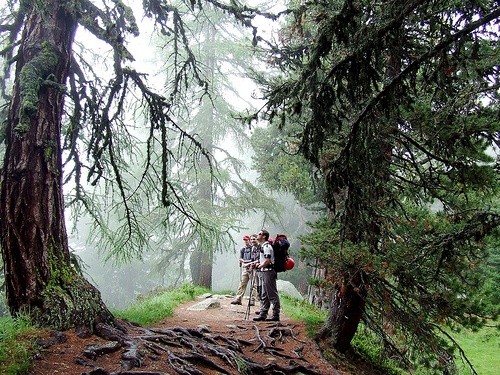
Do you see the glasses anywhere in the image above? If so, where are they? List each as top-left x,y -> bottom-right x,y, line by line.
259,233 -> 262,235
250,239 -> 257,240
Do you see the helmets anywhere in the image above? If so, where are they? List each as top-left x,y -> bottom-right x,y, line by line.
284,258 -> 295,270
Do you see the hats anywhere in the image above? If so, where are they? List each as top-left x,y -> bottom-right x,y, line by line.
243,236 -> 250,240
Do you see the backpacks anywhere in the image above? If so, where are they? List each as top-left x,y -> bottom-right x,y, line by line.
273,234 -> 290,272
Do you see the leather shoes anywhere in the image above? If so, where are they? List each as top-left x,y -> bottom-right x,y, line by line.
253,316 -> 265,321
265,316 -> 279,321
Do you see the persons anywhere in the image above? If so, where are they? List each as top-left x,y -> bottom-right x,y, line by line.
231,236 -> 256,306
253,230 -> 280,321
239,234 -> 262,315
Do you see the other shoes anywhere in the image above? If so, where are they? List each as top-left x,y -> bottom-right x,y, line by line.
231,300 -> 242,305
248,300 -> 255,306
255,312 -> 260,315
